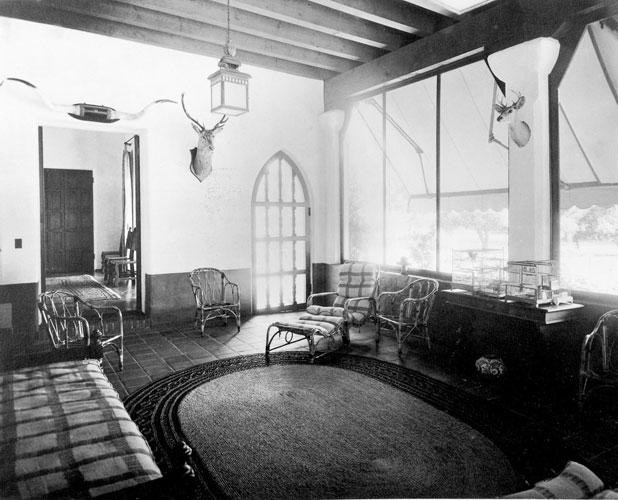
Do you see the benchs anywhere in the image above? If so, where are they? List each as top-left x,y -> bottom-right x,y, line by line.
0,326 -> 205,499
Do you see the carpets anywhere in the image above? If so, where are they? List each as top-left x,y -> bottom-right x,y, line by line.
119,349 -> 560,497
44,272 -> 122,303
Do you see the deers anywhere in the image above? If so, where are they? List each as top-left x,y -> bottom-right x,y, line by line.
180,91 -> 230,180
491,89 -> 533,147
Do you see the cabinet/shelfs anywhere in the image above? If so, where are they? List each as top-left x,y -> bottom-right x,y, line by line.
434,284 -> 588,436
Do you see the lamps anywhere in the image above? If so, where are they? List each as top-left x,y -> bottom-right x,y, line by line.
205,0 -> 252,118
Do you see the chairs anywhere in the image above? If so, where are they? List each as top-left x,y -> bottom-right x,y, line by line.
101,227 -> 137,289
264,258 -> 384,367
187,266 -> 241,338
576,309 -> 618,439
35,289 -> 125,371
373,277 -> 442,360
